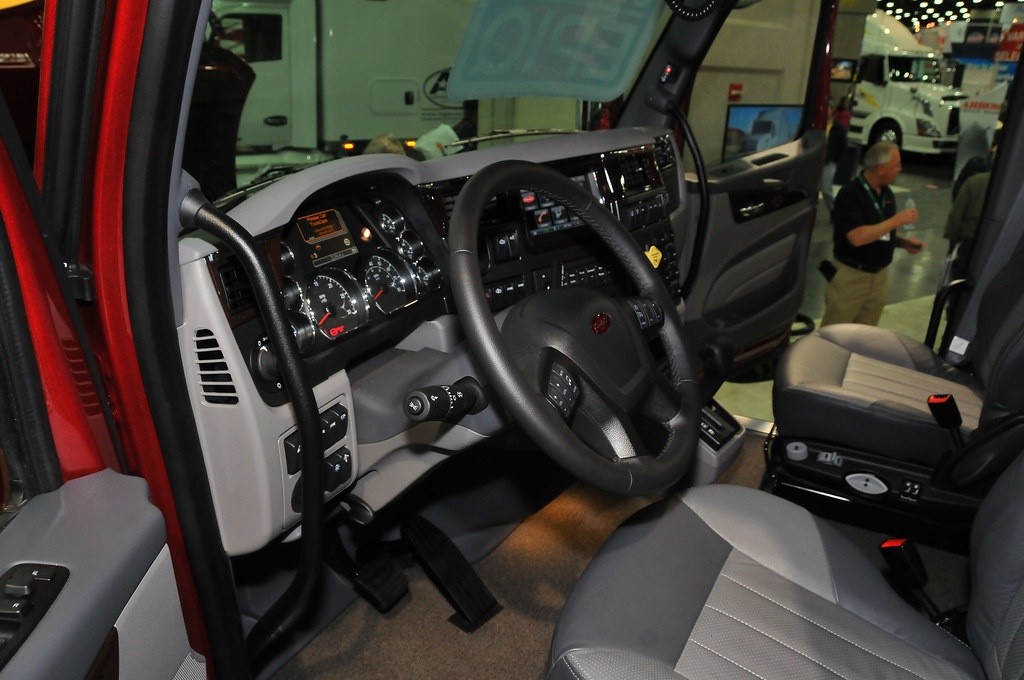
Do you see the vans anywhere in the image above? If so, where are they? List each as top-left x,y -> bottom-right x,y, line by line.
845,11 -> 965,167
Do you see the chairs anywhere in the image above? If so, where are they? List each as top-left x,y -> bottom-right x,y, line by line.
771,252 -> 1024,470
547,448 -> 1024,678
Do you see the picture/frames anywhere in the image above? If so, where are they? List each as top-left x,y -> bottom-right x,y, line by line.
721,104 -> 804,165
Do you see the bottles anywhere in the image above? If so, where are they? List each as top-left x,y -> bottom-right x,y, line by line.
903,195 -> 917,230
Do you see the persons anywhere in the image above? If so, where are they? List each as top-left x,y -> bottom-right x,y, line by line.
817,141 -> 925,332
943,139 -> 1000,268
817,104 -> 846,224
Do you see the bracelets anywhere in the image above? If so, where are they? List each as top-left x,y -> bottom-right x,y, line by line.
887,215 -> 896,230
